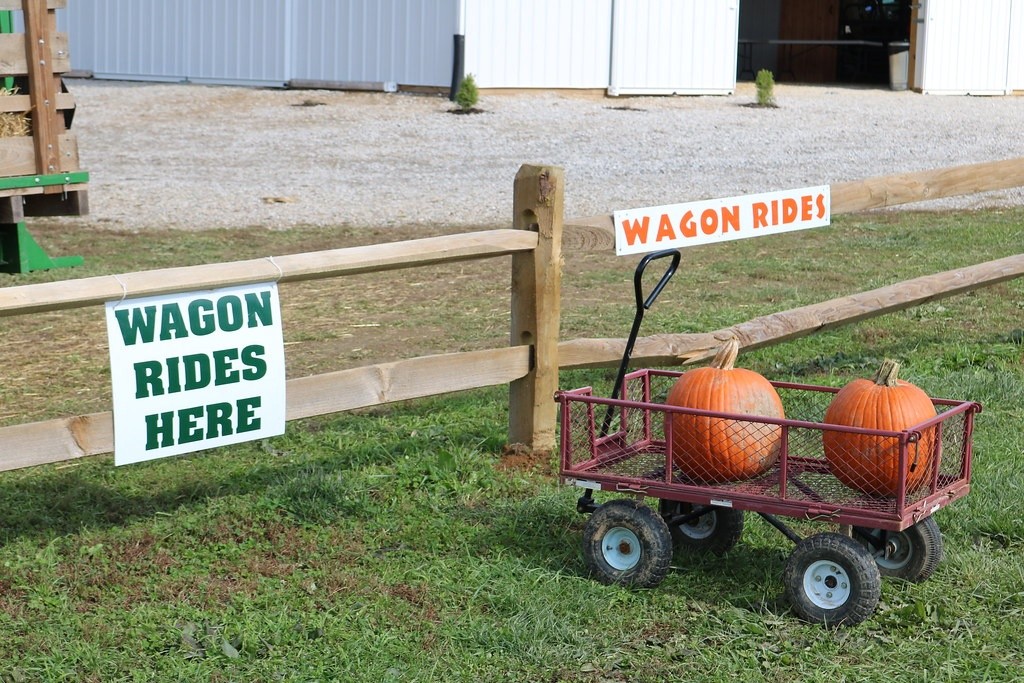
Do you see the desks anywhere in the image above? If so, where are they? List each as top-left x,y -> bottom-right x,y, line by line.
737,39 -> 763,80
769,40 -> 882,82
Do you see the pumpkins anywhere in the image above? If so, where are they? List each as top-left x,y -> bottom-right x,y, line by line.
823,357 -> 937,497
663,337 -> 785,485
